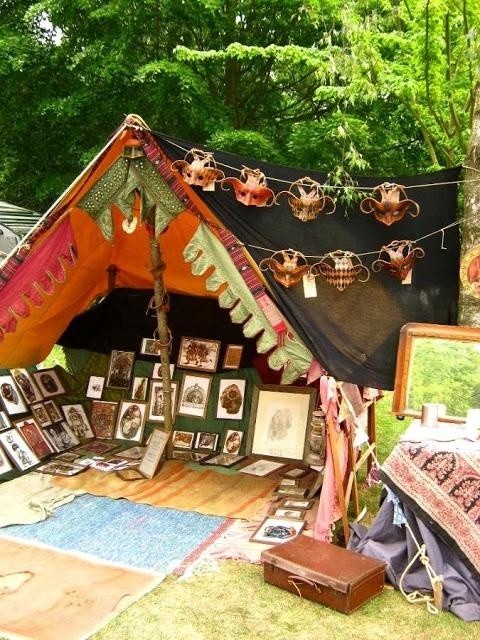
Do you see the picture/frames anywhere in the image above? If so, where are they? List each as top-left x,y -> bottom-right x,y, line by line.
35,440 -> 150,481
237,455 -> 314,543
85,339 -> 245,478
391,320 -> 480,426
250,383 -> 315,461
1,366 -> 96,479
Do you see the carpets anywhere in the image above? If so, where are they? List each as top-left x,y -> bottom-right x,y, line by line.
0,494 -> 243,579
0,535 -> 167,640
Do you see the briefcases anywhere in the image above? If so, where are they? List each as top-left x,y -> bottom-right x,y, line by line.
258,532 -> 389,618
